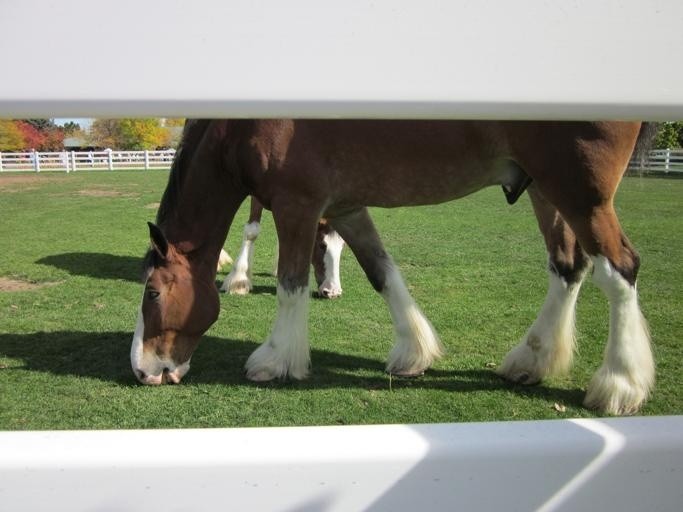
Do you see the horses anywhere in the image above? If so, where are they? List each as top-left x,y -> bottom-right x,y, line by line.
130,118 -> 658,417
216,196 -> 345,298
